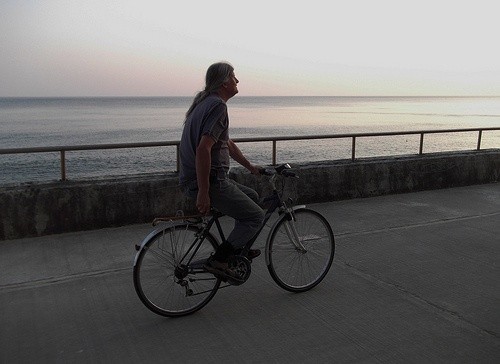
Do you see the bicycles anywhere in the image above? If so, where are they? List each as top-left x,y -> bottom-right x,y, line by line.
133,163 -> 335,319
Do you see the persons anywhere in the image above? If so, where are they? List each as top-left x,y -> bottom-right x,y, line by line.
181,62 -> 266,280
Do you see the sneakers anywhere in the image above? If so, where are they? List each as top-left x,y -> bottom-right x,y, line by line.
236,248 -> 261,260
204,252 -> 243,282
